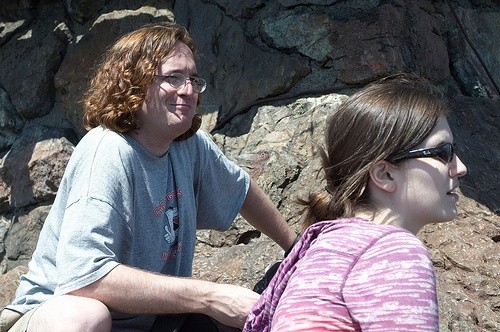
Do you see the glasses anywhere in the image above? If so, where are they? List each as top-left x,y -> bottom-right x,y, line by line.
391,141 -> 457,164
154,73 -> 208,94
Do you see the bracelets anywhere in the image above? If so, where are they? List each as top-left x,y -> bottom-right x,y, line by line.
284,233 -> 301,259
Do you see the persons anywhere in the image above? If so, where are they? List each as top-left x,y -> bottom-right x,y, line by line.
241,72 -> 468,332
0,21 -> 301,332
